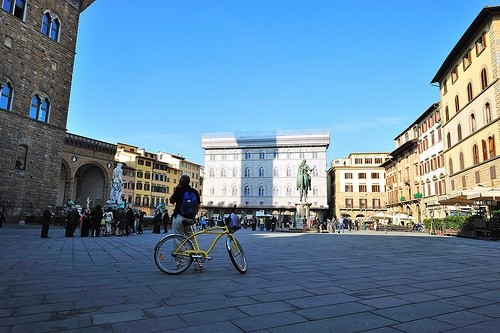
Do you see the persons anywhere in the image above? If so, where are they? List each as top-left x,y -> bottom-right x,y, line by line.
109,163 -> 125,203
311,216 -> 364,234
197,211 -> 293,231
170,175 -> 203,272
296,159 -> 312,191
40,205 -> 53,238
64,204 -> 169,237
303,215 -> 307,233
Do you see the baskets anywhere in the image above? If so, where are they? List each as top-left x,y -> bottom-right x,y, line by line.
221,214 -> 241,233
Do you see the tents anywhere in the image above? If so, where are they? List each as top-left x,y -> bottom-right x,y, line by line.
427,189 -> 500,219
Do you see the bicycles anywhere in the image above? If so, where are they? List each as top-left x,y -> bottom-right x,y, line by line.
153,204 -> 248,275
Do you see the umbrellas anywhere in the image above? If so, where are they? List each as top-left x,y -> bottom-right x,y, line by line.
394,213 -> 415,221
369,212 -> 391,220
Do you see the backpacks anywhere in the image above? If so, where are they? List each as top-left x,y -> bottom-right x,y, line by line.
179,191 -> 197,216
